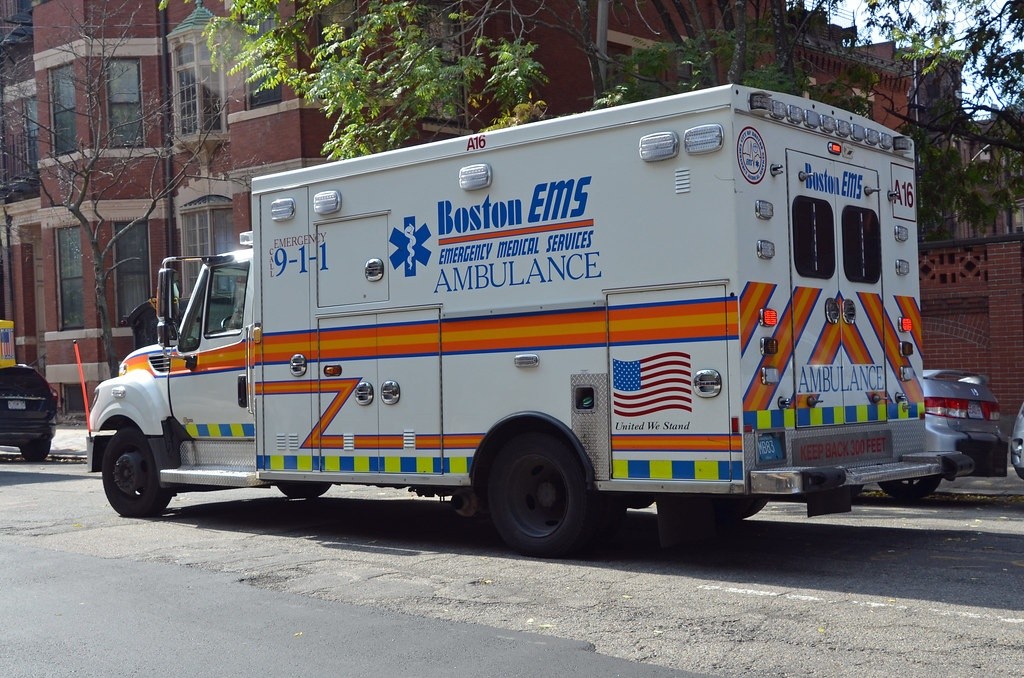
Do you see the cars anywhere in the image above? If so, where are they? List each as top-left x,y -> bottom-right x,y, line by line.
0,363 -> 59,465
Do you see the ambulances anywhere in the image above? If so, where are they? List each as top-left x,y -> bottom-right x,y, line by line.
81,83 -> 975,560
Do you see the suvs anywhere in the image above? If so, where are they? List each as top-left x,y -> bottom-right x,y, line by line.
877,368 -> 1002,501
